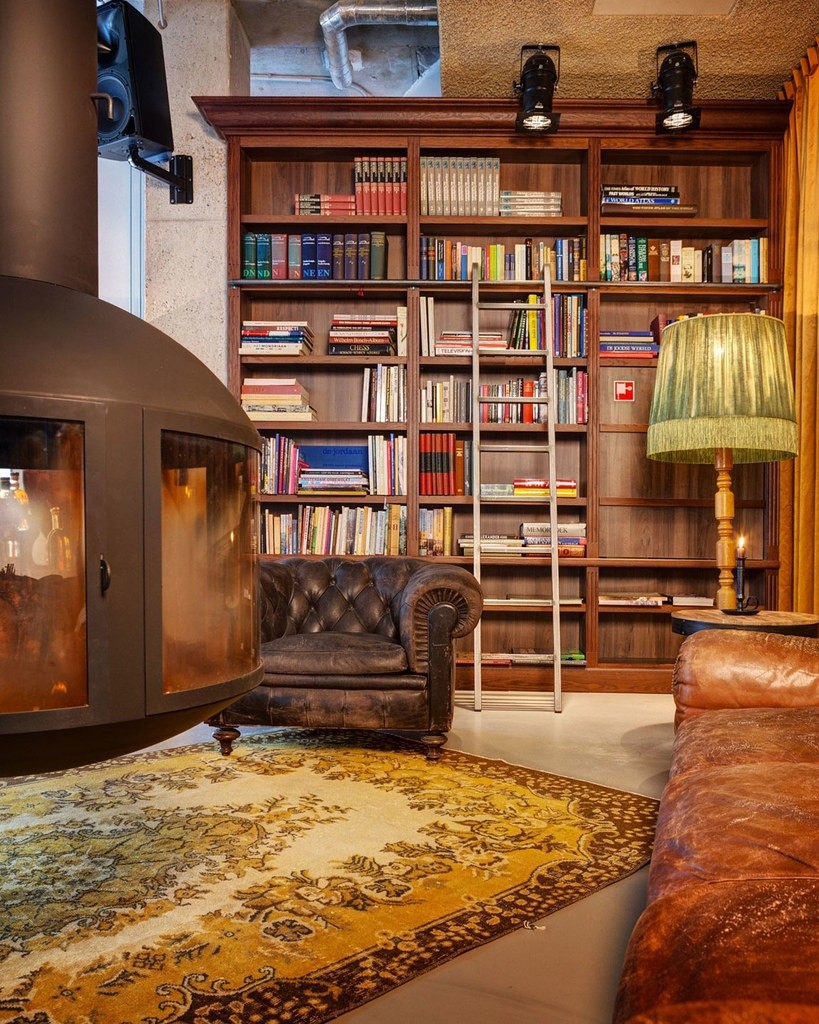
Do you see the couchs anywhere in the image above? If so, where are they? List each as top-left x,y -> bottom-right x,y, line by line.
615,628 -> 819,1024
199,551 -> 487,758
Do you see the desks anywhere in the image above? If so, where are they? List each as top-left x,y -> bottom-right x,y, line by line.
670,612 -> 819,638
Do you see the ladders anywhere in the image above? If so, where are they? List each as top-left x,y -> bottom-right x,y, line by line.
471,264 -> 565,715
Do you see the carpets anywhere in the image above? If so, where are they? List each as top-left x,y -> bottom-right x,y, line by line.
1,730 -> 659,1024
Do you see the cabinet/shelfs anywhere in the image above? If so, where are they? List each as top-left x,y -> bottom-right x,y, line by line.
190,93 -> 793,692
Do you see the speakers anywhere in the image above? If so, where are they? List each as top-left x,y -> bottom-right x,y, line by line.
96,0 -> 174,161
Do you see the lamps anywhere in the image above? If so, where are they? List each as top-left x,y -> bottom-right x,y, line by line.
647,312 -> 798,612
648,39 -> 702,134
512,46 -> 562,130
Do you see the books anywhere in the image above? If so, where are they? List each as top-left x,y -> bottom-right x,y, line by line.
599,302 -> 773,359
418,234 -> 588,283
455,592 -> 716,666
294,156 -> 408,216
238,305 -> 407,359
418,294 -> 589,360
418,506 -> 588,559
257,504 -> 407,558
241,231 -> 408,282
418,432 -> 578,499
598,182 -> 769,284
239,363 -> 407,421
418,156 -> 564,217
254,433 -> 409,498
420,366 -> 589,425
